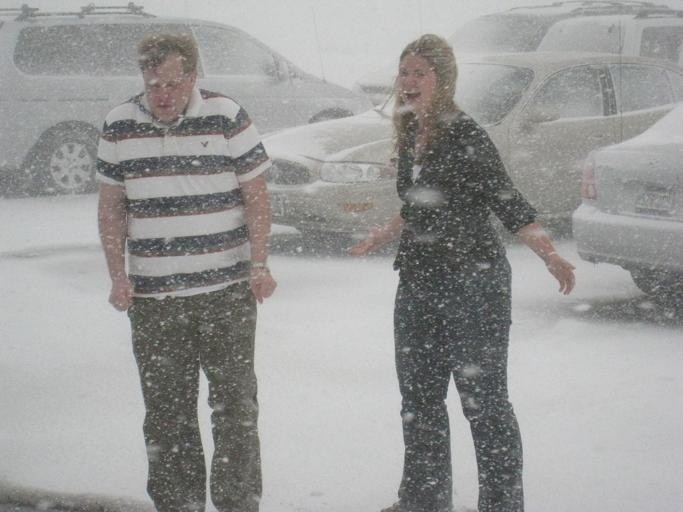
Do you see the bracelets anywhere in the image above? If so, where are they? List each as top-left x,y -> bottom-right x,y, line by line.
250,261 -> 268,270
544,250 -> 557,262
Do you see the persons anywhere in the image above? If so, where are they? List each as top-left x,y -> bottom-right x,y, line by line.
343,32 -> 578,512
94,31 -> 277,511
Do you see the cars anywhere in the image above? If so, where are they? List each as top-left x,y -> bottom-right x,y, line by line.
260,52 -> 683,253
570,104 -> 683,295
444,4 -> 556,56
0,0 -> 374,196
536,5 -> 682,55
358,68 -> 392,111
